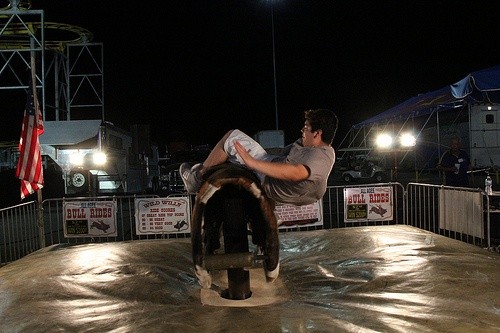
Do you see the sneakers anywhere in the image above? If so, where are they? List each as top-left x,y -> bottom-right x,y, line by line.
180,162 -> 202,195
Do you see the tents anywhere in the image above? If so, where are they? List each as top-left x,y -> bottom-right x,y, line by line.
351,66 -> 500,184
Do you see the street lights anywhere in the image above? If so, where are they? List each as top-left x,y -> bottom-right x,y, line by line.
58,142 -> 117,199
365,122 -> 423,184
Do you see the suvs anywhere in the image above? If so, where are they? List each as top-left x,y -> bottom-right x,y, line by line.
154,148 -> 212,198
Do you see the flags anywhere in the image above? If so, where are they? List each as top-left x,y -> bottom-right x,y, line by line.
15,74 -> 45,201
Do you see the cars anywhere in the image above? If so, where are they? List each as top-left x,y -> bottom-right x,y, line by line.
331,145 -> 392,182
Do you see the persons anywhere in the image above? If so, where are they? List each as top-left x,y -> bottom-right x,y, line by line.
179,110 -> 338,206
438,136 -> 470,187
